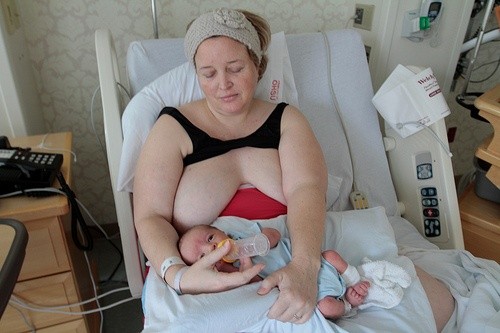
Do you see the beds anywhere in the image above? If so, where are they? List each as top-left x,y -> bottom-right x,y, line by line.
93,28 -> 500,333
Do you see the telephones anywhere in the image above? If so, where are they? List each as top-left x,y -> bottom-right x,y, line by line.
0,136 -> 63,191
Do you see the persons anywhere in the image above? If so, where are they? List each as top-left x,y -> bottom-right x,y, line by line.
131,8 -> 455,332
177,224 -> 370,319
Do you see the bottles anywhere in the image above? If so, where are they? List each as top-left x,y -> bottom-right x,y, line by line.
217,233 -> 270,262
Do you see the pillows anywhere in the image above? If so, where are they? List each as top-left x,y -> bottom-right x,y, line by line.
116,31 -> 297,193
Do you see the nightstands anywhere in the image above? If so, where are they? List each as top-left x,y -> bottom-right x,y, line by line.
0,131 -> 101,333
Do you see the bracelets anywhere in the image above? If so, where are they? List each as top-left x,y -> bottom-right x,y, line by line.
160,256 -> 186,284
174,266 -> 189,295
